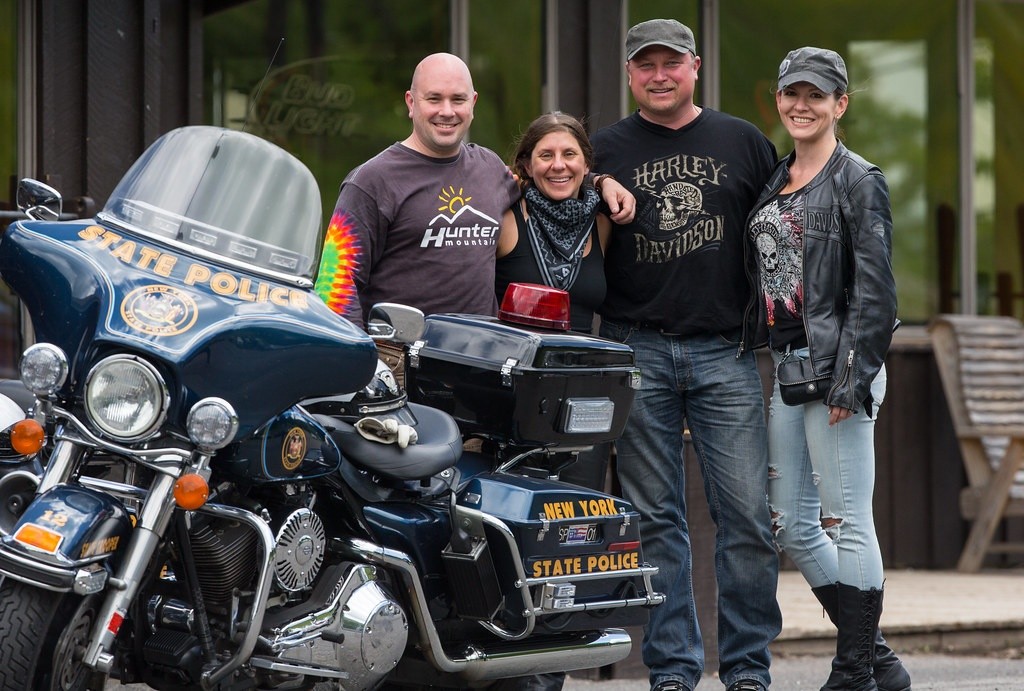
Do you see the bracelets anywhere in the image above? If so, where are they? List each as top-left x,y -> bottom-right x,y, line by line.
594,173 -> 616,195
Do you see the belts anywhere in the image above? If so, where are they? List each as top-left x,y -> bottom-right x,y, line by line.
773,333 -> 808,352
600,316 -> 702,337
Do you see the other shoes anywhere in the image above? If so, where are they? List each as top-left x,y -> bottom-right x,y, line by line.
653,680 -> 690,691
728,679 -> 766,691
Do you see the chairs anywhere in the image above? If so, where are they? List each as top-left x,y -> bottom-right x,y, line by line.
927,315 -> 1024,573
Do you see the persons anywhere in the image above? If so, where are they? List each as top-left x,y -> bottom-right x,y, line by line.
745,46 -> 912,691
313,51 -> 637,387
588,18 -> 783,691
489,112 -> 613,334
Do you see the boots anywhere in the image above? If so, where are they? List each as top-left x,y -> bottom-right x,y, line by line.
811,583 -> 911,691
820,578 -> 887,691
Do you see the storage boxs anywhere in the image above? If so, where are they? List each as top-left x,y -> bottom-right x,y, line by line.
405,314 -> 639,448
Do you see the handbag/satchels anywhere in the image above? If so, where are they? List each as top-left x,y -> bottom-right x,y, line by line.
776,354 -> 832,406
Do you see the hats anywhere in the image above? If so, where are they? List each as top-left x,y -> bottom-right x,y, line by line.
625,19 -> 696,60
777,46 -> 848,94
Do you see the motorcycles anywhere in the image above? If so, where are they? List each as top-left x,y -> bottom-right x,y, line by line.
0,122 -> 669,690
